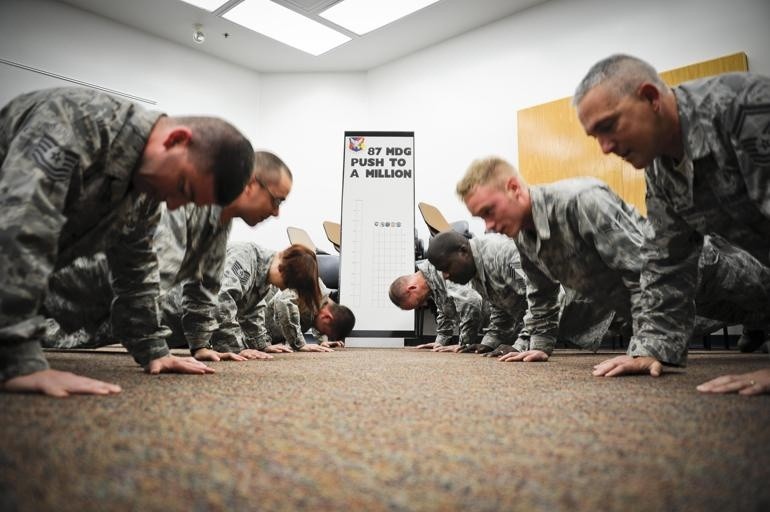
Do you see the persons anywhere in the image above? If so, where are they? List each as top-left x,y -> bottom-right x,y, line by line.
455,157 -> 770,362
571,51 -> 770,397
388,259 -> 490,353
62,241 -> 322,360
2,85 -> 256,400
265,277 -> 355,353
427,231 -> 770,357
39,150 -> 295,363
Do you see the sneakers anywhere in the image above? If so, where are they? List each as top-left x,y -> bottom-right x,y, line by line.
735,325 -> 770,353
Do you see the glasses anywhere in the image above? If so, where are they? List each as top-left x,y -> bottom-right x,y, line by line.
256,177 -> 285,208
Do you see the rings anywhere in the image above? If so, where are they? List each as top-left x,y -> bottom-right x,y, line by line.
749,378 -> 755,386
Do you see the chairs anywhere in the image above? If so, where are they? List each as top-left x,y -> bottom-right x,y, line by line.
286,201 -> 472,347
